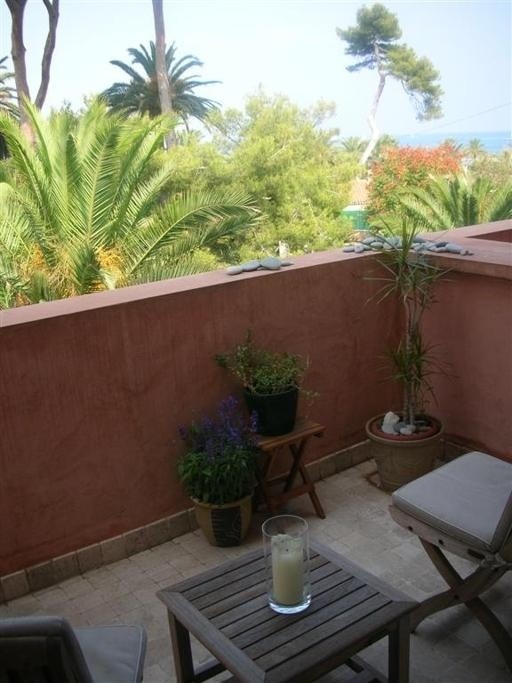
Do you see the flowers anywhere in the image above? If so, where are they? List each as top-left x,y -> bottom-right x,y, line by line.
172,391 -> 259,503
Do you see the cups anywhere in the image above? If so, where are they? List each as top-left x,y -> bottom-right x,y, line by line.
260,512 -> 313,615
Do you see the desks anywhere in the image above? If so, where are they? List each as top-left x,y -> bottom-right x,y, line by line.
156,532 -> 420,683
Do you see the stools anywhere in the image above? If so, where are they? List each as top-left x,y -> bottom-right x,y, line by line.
252,416 -> 326,534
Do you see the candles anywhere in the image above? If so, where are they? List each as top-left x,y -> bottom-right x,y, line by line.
271,534 -> 304,605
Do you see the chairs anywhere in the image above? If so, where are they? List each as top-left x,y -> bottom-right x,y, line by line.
389,451 -> 512,671
0,616 -> 147,683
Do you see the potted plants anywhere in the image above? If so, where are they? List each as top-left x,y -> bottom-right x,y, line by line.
344,213 -> 461,496
209,329 -> 318,435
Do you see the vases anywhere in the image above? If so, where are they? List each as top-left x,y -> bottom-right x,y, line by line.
189,488 -> 255,547
